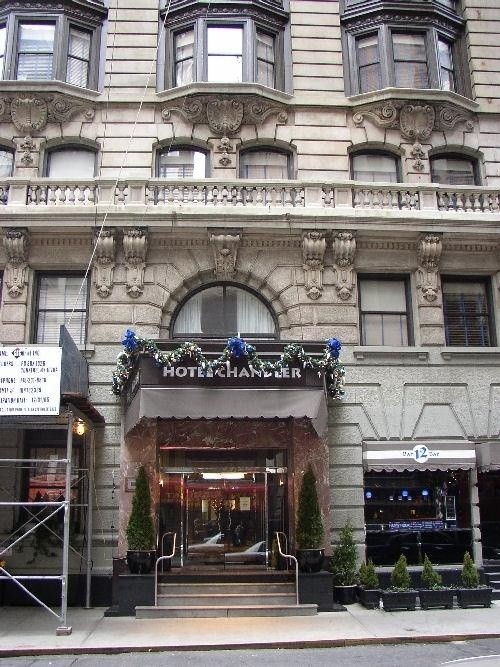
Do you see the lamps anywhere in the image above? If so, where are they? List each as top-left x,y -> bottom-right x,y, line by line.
77,418 -> 85,436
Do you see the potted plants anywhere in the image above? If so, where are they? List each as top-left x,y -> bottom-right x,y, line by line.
125,465 -> 157,574
294,462 -> 326,573
331,517 -> 493,609
